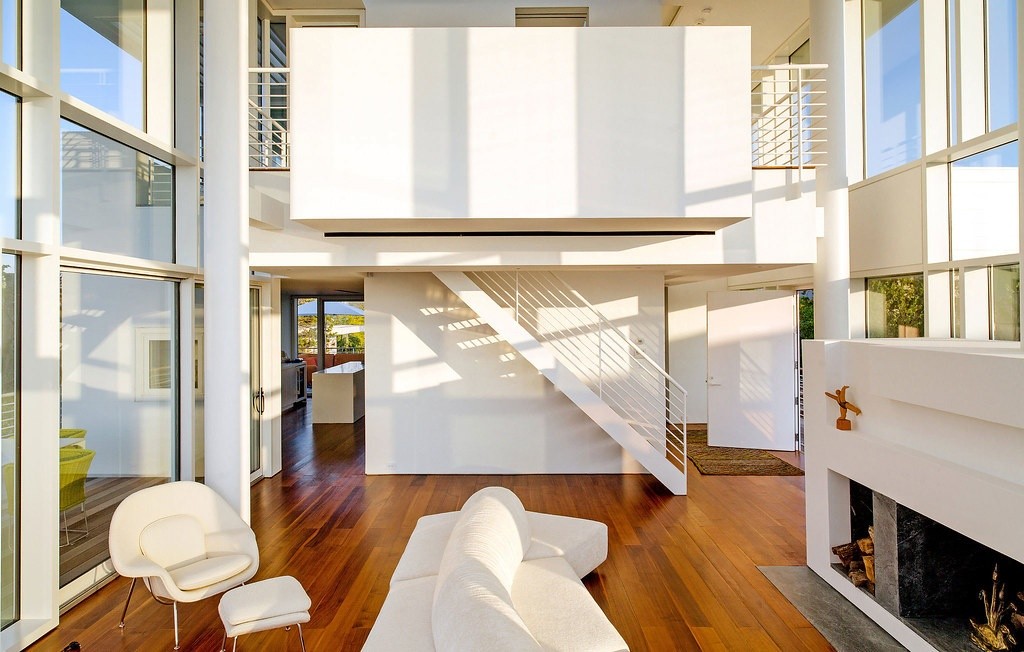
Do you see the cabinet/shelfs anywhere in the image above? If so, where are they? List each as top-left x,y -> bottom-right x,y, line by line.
281,361 -> 308,413
312,361 -> 365,424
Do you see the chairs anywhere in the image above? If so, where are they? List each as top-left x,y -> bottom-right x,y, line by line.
7,449 -> 95,551
109,479 -> 259,649
59,428 -> 87,513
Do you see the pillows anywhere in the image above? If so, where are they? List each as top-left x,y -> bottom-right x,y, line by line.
220,575 -> 312,626
306,357 -> 317,366
168,554 -> 251,591
138,514 -> 206,572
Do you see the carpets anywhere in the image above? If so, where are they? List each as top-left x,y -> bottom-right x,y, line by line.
666,429 -> 805,476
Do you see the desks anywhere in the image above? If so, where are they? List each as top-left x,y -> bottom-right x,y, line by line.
0,437 -> 84,559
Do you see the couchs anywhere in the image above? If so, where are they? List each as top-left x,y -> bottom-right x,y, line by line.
360,486 -> 630,652
298,354 -> 364,388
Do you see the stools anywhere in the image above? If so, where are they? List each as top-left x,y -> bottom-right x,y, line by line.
220,574 -> 311,652
